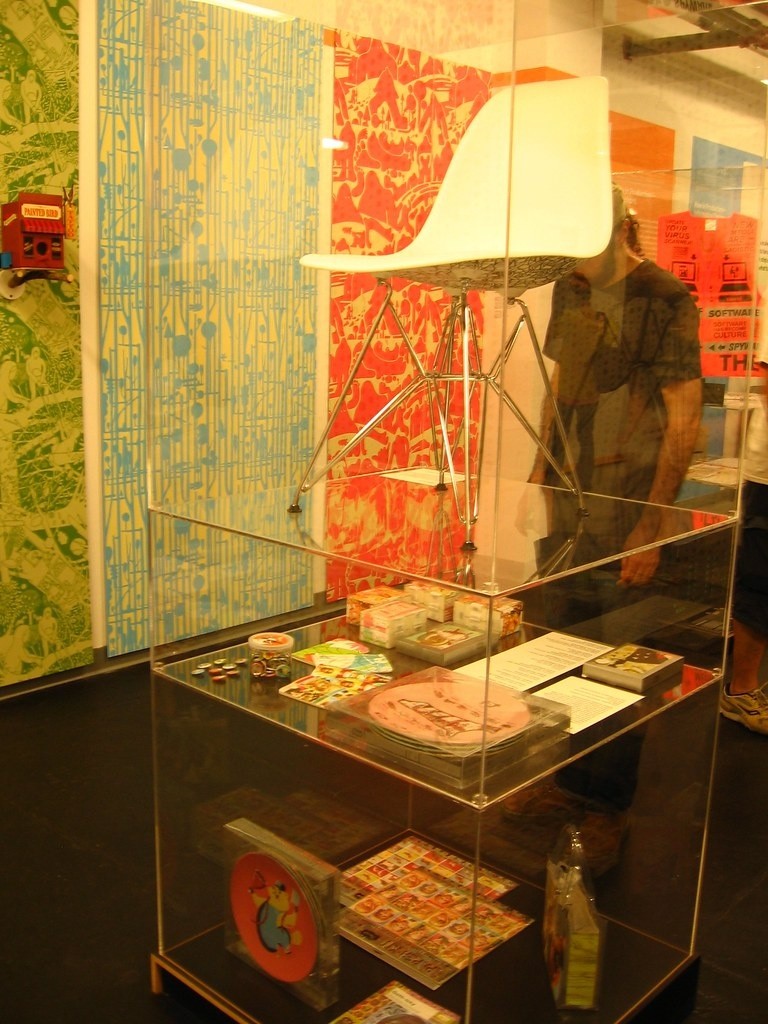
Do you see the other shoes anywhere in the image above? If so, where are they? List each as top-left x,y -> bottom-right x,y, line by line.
500,781 -> 592,821
575,808 -> 631,884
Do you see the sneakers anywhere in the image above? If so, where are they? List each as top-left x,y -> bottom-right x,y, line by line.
718,681 -> 768,736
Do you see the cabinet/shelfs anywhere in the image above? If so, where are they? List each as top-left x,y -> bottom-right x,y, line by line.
145,466 -> 737,1024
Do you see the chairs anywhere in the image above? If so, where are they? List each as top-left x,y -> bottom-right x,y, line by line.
286,77 -> 614,551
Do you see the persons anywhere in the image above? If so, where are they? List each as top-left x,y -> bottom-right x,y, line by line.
503,183 -> 704,899
